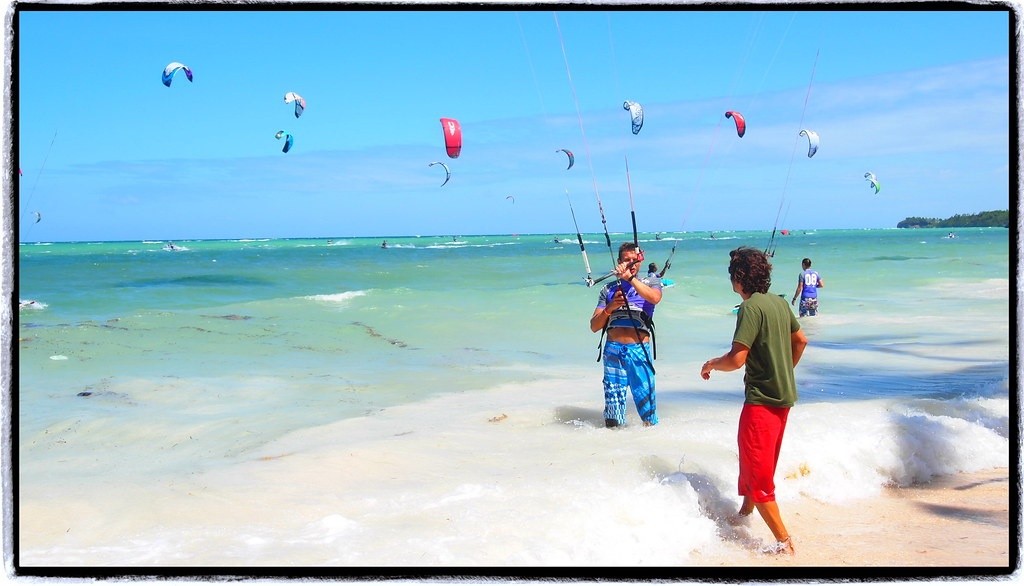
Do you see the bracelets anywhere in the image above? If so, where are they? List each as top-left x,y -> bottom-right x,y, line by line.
628,275 -> 635,282
603,308 -> 611,316
793,298 -> 796,300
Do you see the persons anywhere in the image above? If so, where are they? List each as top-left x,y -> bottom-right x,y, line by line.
648,262 -> 670,280
701,248 -> 808,555
792,258 -> 824,317
590,242 -> 662,429
